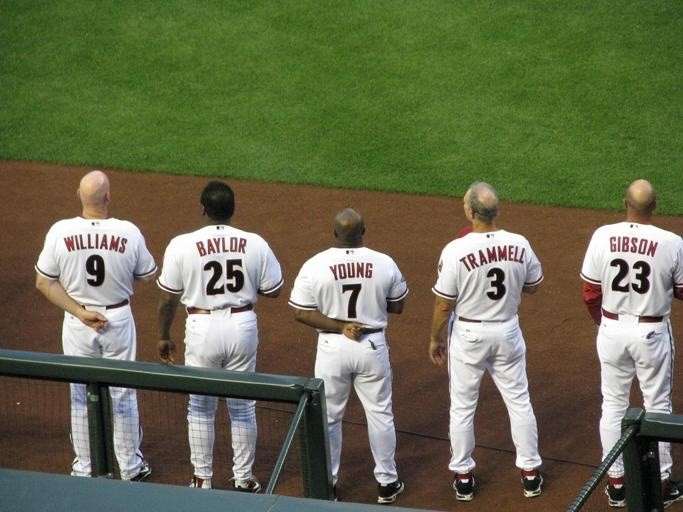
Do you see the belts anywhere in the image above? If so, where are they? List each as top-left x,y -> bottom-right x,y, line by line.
600,308 -> 665,324
185,303 -> 252,314
459,316 -> 482,324
321,326 -> 383,334
81,299 -> 129,310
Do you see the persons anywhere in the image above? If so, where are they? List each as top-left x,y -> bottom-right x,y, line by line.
581,180 -> 683,509
429,182 -> 544,502
155,181 -> 285,491
289,207 -> 409,503
35,171 -> 158,480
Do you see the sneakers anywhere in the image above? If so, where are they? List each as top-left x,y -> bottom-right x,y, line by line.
186,476 -> 213,490
519,471 -> 544,498
451,475 -> 477,503
332,484 -> 340,501
234,479 -> 263,494
661,479 -> 683,510
128,462 -> 152,483
375,478 -> 405,505
603,484 -> 628,507
